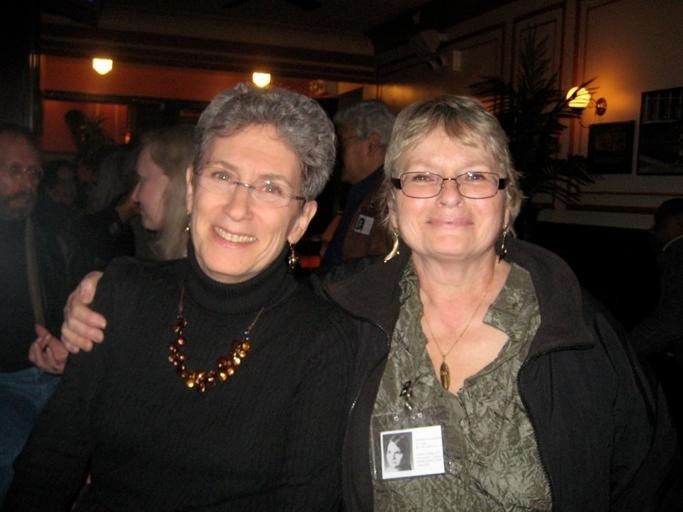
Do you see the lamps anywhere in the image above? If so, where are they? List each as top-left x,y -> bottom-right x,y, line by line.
565,86 -> 612,122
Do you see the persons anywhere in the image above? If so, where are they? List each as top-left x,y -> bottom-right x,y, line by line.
2,109 -> 196,478
289,97 -> 406,287
386,434 -> 408,472
355,216 -> 365,230
0,82 -> 361,508
642,195 -> 682,364
61,97 -> 672,512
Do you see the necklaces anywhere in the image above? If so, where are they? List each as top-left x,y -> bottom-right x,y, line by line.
416,256 -> 507,391
168,280 -> 270,393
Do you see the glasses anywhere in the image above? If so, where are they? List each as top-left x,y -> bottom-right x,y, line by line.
392,170 -> 510,200
192,165 -> 305,208
